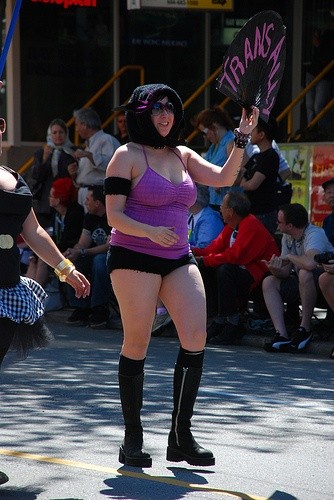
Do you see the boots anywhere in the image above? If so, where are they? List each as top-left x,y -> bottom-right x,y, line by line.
166,362 -> 217,467
118,373 -> 156,467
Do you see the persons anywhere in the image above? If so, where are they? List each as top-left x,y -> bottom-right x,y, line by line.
0,119 -> 91,368
152,107 -> 334,353
17,108 -> 128,328
103,85 -> 260,466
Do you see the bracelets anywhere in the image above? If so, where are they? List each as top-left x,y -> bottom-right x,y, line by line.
80,246 -> 86,257
234,136 -> 248,148
54,259 -> 73,275
234,128 -> 251,139
58,264 -> 76,282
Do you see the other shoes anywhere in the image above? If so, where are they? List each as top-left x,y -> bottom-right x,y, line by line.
291,330 -> 314,351
206,320 -> 248,346
86,315 -> 110,327
64,309 -> 88,324
264,336 -> 291,352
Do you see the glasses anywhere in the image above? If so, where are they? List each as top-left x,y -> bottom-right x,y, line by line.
232,225 -> 239,239
150,101 -> 175,116
200,126 -> 209,136
